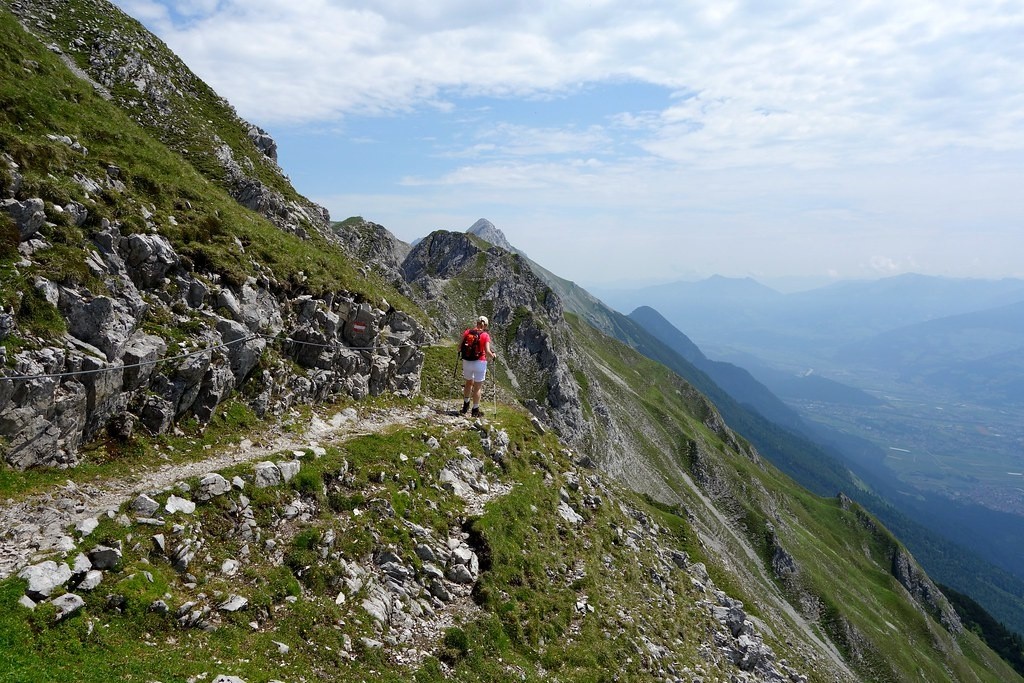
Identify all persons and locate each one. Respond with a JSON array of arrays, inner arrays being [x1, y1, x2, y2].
[[457, 316, 495, 418]]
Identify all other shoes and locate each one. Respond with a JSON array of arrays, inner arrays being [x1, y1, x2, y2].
[[472, 407, 484, 418], [460, 400, 470, 414]]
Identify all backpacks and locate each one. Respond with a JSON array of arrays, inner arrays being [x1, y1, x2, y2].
[[461, 330, 480, 361]]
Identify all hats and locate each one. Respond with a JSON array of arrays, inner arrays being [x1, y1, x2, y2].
[[477, 316, 488, 325]]
